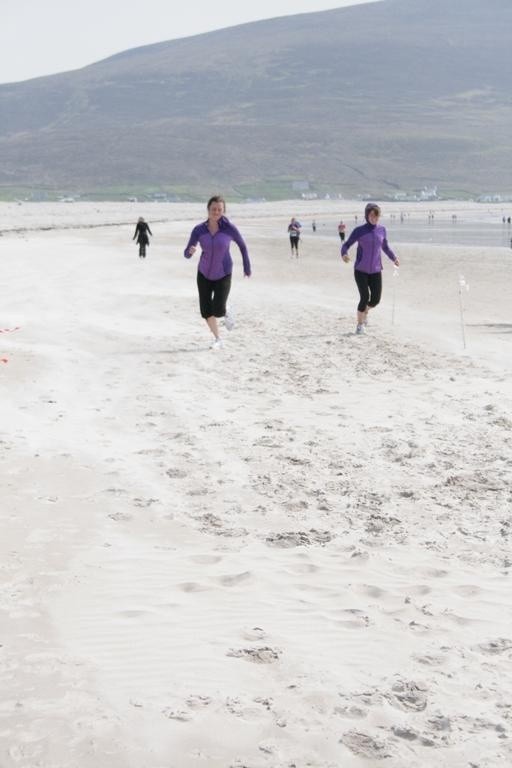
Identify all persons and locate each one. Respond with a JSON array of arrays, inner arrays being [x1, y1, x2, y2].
[[132, 216, 153, 258], [183, 194, 252, 352], [287, 217, 302, 260], [312, 219, 317, 232], [295, 219, 303, 244], [341, 203, 401, 335], [338, 221, 347, 244]]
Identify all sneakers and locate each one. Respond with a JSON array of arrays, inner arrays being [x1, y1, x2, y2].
[[208, 336, 224, 351], [355, 319, 370, 335]]
